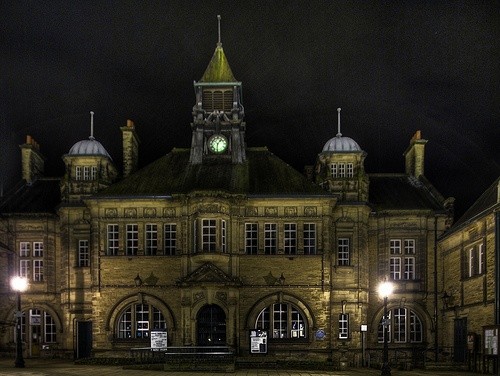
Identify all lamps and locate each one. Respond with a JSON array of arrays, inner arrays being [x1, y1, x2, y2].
[[134, 273, 142, 286], [441, 290, 451, 303], [279, 273, 285, 285]]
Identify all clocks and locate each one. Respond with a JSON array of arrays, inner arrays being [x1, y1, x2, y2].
[[207, 133, 228, 154]]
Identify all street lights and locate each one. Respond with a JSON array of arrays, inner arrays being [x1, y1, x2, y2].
[[12, 277, 26, 367], [379, 277, 392, 374]]
